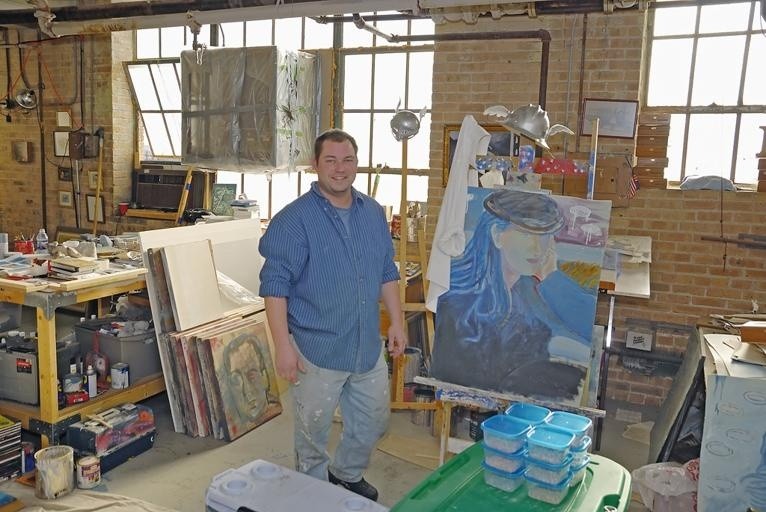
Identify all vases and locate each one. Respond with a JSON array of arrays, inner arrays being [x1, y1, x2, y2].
[[580, 98, 639, 139]]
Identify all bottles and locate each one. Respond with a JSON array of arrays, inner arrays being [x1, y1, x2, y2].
[[36, 228, 48, 254]]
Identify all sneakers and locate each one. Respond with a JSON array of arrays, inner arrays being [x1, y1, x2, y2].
[[326, 466, 379, 502]]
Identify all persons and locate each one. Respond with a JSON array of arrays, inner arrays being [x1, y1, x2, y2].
[[430, 190, 598, 403], [259, 128, 405, 502], [223, 333, 282, 439]]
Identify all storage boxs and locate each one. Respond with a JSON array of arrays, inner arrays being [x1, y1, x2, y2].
[[382, 432, 634, 512]]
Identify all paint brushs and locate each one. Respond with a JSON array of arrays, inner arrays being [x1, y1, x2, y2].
[[12, 232, 36, 241]]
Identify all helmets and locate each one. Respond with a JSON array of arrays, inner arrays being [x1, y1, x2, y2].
[[494, 103, 552, 152], [390, 109, 420, 142]]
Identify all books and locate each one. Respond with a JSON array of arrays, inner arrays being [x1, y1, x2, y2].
[[50, 256, 98, 276]]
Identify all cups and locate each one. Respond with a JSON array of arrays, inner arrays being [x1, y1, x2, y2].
[[118, 203, 129, 215]]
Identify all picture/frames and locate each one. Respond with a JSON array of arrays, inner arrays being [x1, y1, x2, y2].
[[85, 170, 105, 224], [442, 123, 511, 185], [53, 131, 73, 209]]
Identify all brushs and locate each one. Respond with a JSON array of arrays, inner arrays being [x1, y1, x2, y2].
[[85, 414, 113, 429]]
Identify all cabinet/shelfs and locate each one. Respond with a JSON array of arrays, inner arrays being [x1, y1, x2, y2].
[[2, 245, 167, 450]]
